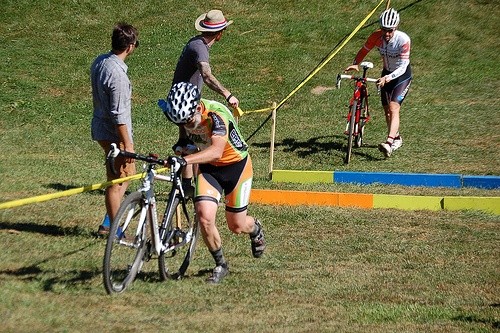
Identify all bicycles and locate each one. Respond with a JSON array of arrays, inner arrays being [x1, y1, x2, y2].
[[336, 61, 381, 164], [101, 143, 199, 297]]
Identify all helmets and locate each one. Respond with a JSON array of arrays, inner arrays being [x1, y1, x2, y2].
[[164, 82, 201, 125], [380, 8, 400, 27]]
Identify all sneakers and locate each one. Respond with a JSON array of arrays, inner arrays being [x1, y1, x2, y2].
[[249, 218, 265, 257], [378, 142, 392, 158], [391, 137, 402, 150], [207, 263, 229, 283]]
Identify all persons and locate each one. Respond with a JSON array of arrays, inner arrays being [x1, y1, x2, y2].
[[173, 10, 239, 200], [345, 8, 413, 158], [165, 82, 265, 283], [89, 22, 140, 239]]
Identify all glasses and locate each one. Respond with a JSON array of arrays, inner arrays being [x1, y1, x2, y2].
[[126, 40, 140, 48], [381, 28, 397, 33], [176, 119, 196, 127]]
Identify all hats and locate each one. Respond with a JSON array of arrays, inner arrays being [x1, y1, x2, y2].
[[195, 9, 233, 32]]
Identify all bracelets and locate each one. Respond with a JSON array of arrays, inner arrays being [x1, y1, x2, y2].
[[226, 94, 233, 102]]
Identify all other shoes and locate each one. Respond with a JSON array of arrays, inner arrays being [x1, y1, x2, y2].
[[114, 231, 137, 244], [97, 225, 110, 238]]
